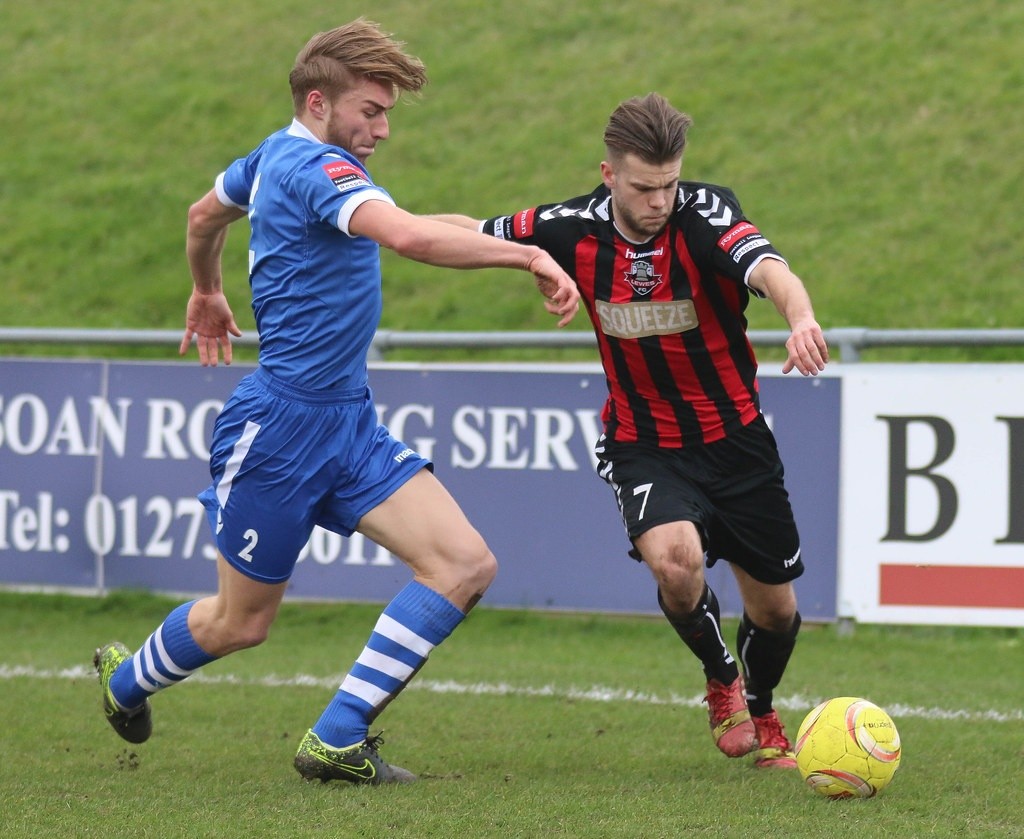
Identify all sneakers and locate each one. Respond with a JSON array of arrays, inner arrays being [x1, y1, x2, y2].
[[293, 728, 415, 784], [700, 673, 755, 758], [96, 642, 153, 744], [752, 709, 797, 770]]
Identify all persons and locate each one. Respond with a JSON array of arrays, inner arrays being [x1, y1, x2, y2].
[[416, 91, 830, 768], [93, 16, 581, 788]]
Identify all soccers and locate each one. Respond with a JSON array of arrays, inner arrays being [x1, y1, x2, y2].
[[794, 696, 903, 801]]
